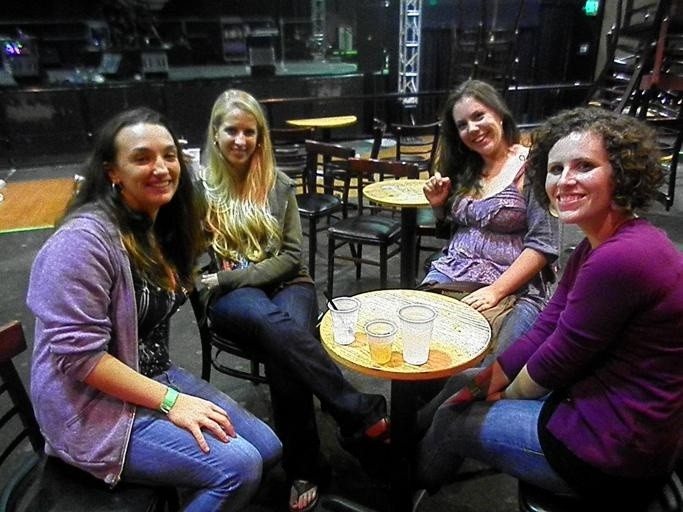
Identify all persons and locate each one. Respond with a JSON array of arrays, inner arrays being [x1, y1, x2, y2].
[[422, 79, 562, 368], [188, 89, 391, 511], [29, 106, 284, 512], [417, 107, 683, 496]]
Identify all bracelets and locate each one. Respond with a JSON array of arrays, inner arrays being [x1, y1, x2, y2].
[[465, 380, 486, 402], [157, 383, 180, 416]]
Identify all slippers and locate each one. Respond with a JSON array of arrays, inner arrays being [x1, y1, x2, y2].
[[287, 479, 319, 511]]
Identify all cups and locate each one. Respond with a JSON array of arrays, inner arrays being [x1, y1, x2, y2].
[[328, 294, 360, 347], [397, 302, 438, 367], [363, 320, 398, 366]]
